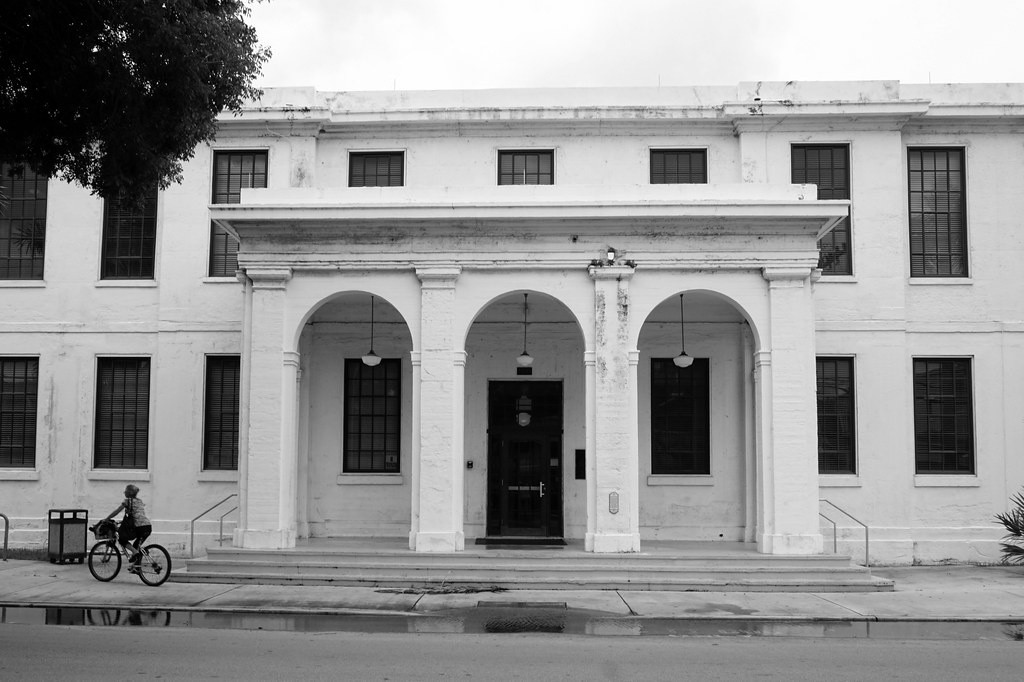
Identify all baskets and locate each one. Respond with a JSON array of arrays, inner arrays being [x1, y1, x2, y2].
[[95, 529, 109, 539]]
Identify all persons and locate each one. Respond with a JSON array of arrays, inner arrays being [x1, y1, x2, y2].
[[106, 484, 152, 571]]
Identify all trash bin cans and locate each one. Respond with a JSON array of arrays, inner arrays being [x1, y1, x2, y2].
[[47, 507, 90, 566]]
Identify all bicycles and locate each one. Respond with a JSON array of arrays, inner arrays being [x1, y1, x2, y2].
[[86, 518, 172, 588]]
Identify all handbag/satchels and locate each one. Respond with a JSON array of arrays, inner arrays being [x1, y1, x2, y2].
[[120, 497, 137, 540]]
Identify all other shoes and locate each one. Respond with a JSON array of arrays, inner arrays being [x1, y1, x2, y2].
[[128, 567, 139, 571], [129, 552, 140, 563]]
